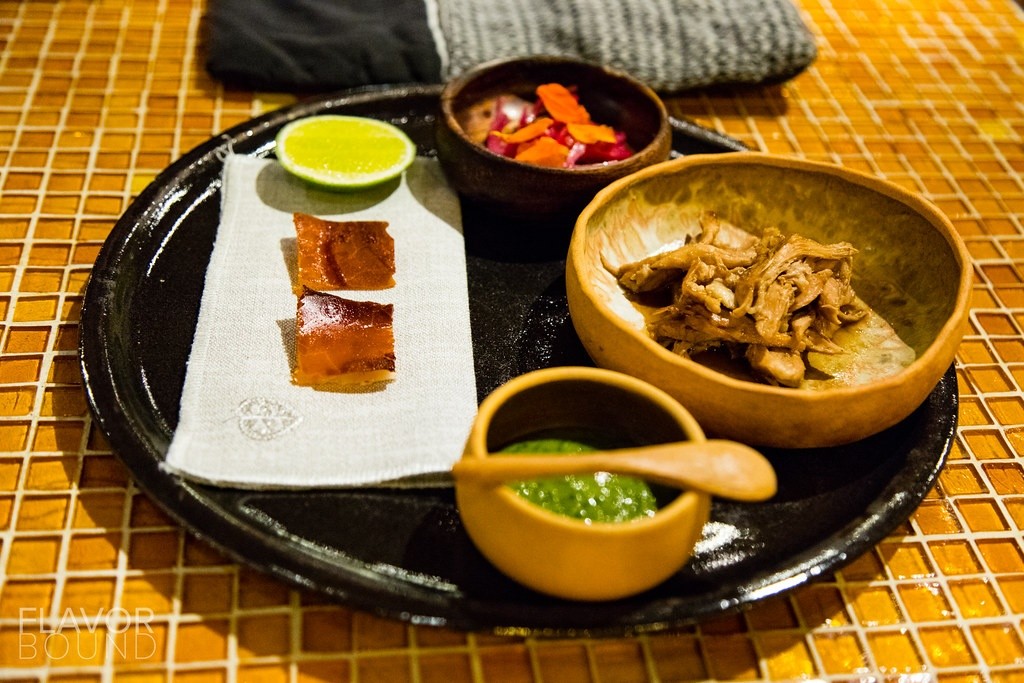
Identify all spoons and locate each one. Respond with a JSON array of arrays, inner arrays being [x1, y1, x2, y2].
[[452, 438, 777, 502]]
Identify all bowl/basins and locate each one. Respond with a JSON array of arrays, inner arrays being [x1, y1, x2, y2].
[[565, 154, 971, 451], [433, 54, 673, 220], [452, 368, 711, 602]]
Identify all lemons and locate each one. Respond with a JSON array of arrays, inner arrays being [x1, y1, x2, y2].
[[275, 114, 417, 189]]
